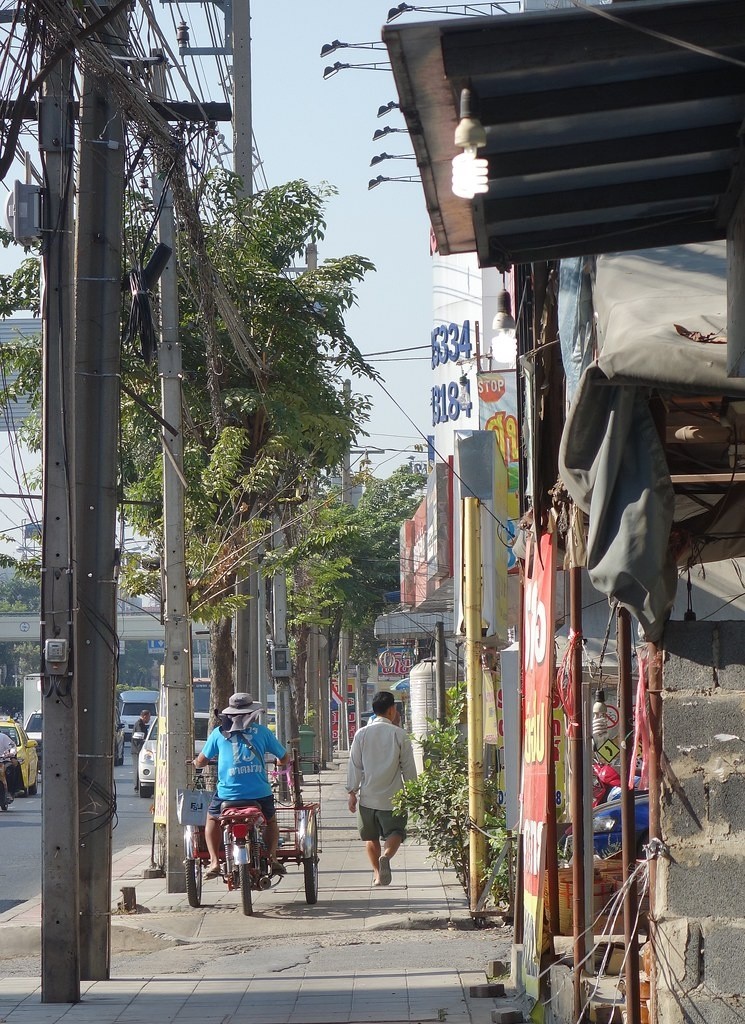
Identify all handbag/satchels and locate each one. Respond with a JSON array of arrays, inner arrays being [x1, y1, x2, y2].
[[176, 775, 215, 826]]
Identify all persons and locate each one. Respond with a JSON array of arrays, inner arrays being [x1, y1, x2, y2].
[[131, 710, 151, 792], [0, 732, 17, 802], [345, 691, 419, 887], [192, 693, 289, 880]]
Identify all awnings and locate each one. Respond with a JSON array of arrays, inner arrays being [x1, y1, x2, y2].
[[390, 678, 410, 691]]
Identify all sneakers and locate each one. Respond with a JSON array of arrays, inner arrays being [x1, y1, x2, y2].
[[373, 853, 392, 886]]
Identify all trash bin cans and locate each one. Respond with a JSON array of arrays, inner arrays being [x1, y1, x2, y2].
[[298, 731, 316, 775]]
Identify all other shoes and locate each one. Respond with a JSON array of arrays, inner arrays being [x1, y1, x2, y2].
[[134, 787, 138, 791]]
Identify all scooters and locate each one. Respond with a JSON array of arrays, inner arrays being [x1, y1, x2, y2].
[[0, 742, 25, 811]]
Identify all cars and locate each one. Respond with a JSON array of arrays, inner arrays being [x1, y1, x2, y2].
[[133, 711, 209, 797], [25, 708, 42, 773], [113, 706, 123, 765], [265, 708, 277, 736], [0, 715, 39, 795]]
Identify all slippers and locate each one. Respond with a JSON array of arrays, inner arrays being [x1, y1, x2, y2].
[[269, 857, 288, 875], [204, 866, 219, 880]]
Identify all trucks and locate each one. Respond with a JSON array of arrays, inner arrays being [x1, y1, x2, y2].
[[118, 691, 159, 741]]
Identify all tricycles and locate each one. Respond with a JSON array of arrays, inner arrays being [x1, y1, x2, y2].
[[180, 746, 321, 916]]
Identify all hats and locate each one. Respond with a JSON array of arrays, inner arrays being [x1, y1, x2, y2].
[[222, 692, 263, 714]]
